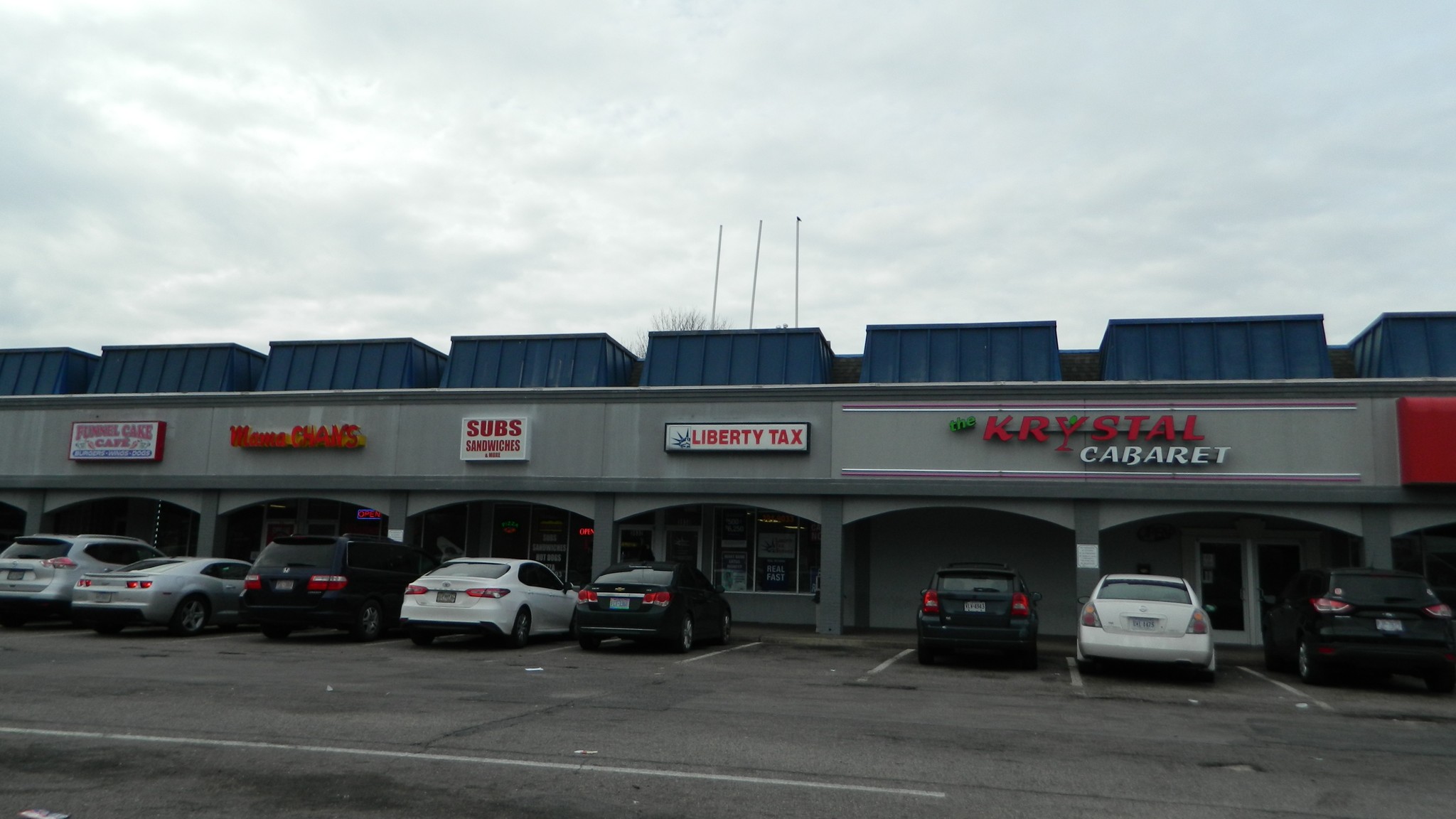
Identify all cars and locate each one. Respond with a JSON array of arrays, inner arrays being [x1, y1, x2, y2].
[[570, 561, 733, 652], [400, 557, 579, 647], [1268, 568, 1456, 688], [71, 557, 253, 637], [1075, 574, 1221, 679]]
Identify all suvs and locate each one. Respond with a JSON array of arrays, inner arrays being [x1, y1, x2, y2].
[[240, 530, 444, 644], [0, 534, 173, 629], [915, 561, 1043, 668]]
